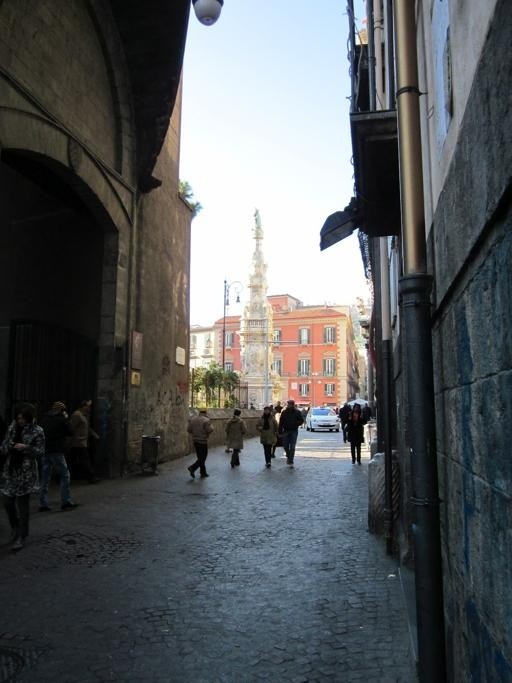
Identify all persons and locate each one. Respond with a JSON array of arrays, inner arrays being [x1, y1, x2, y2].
[[278, 399, 305, 469], [255, 405, 280, 469], [185, 407, 216, 478], [224, 407, 250, 469], [0, 402, 48, 552], [266, 400, 310, 431], [37, 397, 82, 512], [69, 397, 104, 486], [331, 402, 374, 443], [344, 403, 367, 466]]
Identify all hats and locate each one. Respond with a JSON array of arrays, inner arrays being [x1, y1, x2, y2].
[[49, 399, 66, 415]]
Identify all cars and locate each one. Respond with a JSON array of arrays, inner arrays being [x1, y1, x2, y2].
[[304, 406, 340, 433]]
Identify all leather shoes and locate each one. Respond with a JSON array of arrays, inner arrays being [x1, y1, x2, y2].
[[9, 527, 26, 550], [39, 507, 54, 512], [61, 499, 80, 511], [188, 466, 195, 478], [200, 472, 209, 476]]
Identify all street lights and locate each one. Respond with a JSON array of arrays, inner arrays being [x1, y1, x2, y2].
[[222, 278, 245, 370], [190, 0, 225, 26]]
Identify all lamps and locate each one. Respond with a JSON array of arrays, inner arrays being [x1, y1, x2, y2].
[[192, 0, 223, 25]]
